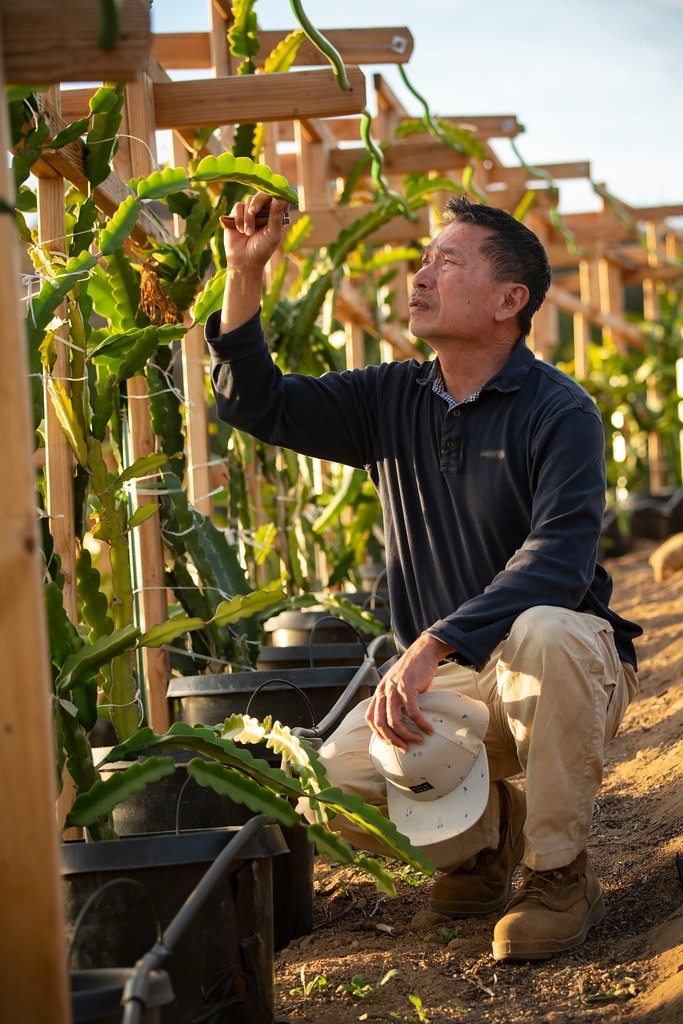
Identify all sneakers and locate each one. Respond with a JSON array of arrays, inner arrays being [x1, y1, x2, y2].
[[491, 848, 607, 961], [427, 779, 527, 918]]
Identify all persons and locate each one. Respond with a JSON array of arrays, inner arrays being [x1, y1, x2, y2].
[[204, 191, 643, 962]]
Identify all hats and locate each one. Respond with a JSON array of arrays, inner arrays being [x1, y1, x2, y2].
[[369, 690, 489, 846]]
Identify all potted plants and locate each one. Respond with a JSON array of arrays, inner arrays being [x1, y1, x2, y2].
[[9, 73, 299, 1024], [139, 370, 392, 670], [154, 471, 381, 773], [71, 256, 422, 948], [260, 472, 386, 647], [315, 545, 377, 612]]
